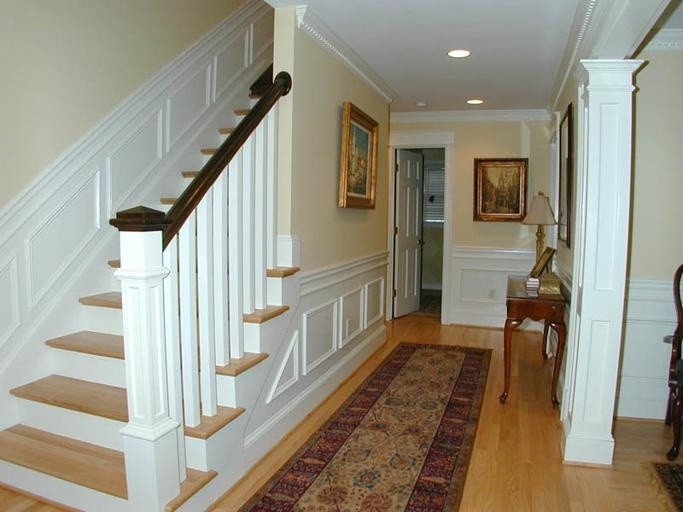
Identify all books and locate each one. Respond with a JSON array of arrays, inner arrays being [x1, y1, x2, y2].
[[524, 277, 539, 297]]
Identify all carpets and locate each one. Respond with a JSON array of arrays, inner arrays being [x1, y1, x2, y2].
[[235, 339, 493, 511], [643, 461, 683, 511]]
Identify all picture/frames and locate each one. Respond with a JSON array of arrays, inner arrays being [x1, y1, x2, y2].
[[529, 246, 555, 279], [556, 103, 573, 249], [337, 99, 378, 211], [472, 157, 528, 223]]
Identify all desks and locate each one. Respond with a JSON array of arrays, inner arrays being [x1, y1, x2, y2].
[[499, 272, 566, 410]]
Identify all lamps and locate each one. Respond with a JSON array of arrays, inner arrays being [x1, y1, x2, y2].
[[521, 190, 556, 263]]
